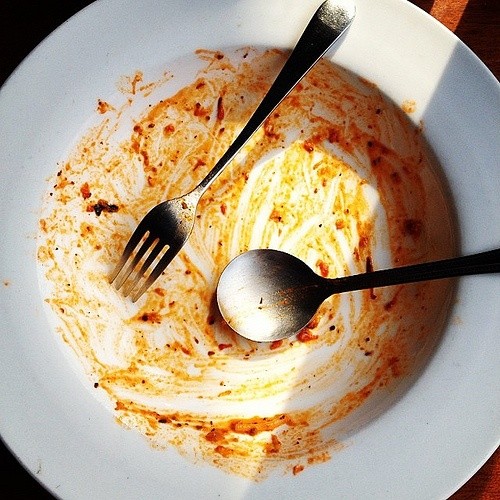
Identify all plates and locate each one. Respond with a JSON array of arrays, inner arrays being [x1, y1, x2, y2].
[[0, 0, 500, 500]]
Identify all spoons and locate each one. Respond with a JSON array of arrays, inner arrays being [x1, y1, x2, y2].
[[216, 249, 500, 342]]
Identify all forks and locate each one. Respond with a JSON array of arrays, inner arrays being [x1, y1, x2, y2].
[[109, 0, 357, 304]]
